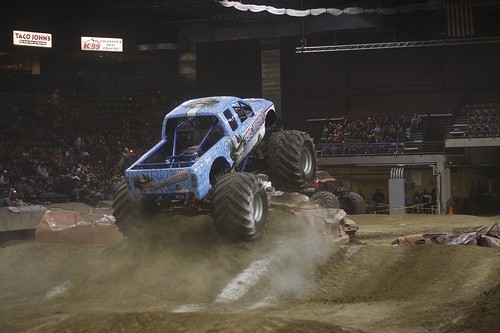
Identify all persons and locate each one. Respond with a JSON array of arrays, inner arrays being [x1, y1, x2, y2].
[[0, 87, 188, 208], [357, 188, 386, 214], [414, 188, 437, 214], [320, 110, 423, 154]]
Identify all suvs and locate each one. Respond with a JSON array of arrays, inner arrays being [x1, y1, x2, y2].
[[304, 170, 366, 217], [111, 96, 318, 242]]
